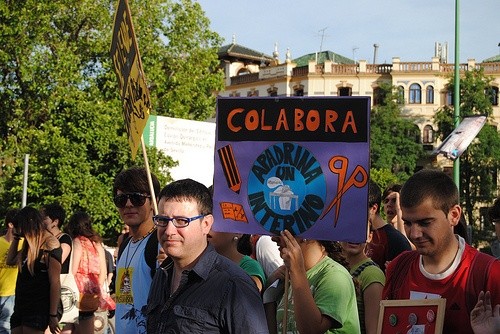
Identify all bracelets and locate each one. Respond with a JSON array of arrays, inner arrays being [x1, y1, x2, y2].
[[49, 314, 57, 317]]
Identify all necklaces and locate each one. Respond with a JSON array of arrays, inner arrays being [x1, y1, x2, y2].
[[55, 232, 61, 237], [125, 222, 158, 276]]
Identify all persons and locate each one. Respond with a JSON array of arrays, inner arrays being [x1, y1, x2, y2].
[[248, 185, 500, 334], [0, 209, 24, 334], [145, 179, 269, 334], [42, 203, 80, 334], [273, 230, 361, 334], [206, 183, 266, 295], [113, 166, 162, 334], [381, 168, 500, 334], [65, 208, 132, 334], [6, 206, 63, 334]]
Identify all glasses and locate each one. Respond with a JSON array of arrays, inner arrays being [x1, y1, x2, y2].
[[152, 212, 212, 228], [113, 193, 150, 209]]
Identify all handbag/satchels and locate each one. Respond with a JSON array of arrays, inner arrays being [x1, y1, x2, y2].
[[75, 292, 99, 311], [59, 273, 80, 325]]
